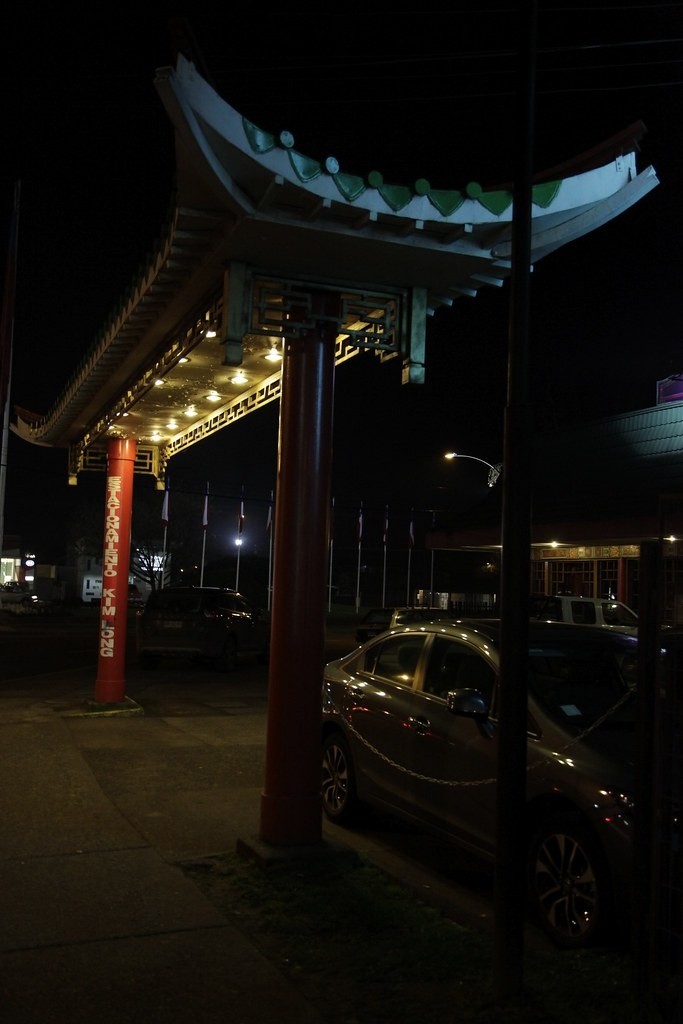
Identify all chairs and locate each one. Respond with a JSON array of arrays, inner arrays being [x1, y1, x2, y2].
[[397, 647, 420, 670]]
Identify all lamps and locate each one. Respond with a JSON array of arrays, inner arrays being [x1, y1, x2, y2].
[[442, 451, 495, 473]]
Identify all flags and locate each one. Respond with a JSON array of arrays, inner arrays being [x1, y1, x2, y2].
[[200, 484, 211, 529], [407, 510, 416, 549], [353, 512, 365, 547], [236, 486, 246, 532], [265, 492, 273, 537], [162, 475, 173, 529], [382, 510, 390, 544], [329, 504, 336, 544]]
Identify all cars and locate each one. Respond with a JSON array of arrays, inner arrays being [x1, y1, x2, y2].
[[134, 586, 272, 673], [0, 581, 49, 611], [320, 617, 683, 956]]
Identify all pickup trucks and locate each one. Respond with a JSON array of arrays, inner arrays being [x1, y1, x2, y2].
[[531, 594, 670, 642]]
